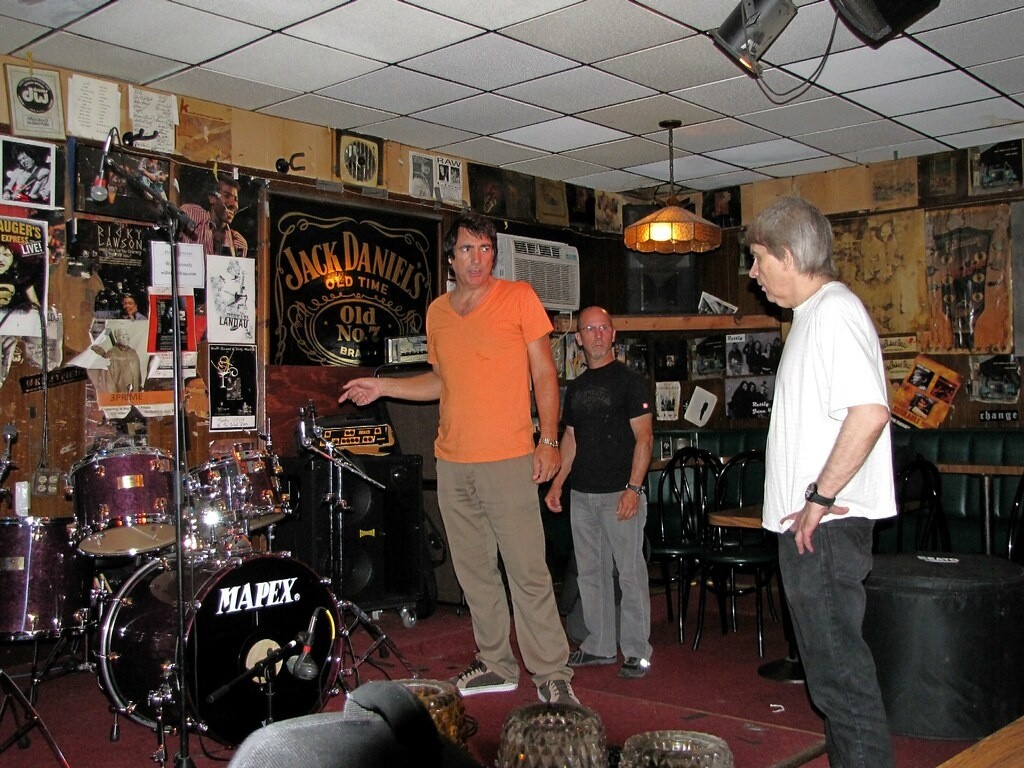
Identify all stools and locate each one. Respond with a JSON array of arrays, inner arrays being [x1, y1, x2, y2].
[[862, 552, 1024, 740]]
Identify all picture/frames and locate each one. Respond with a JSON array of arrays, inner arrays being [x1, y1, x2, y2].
[[2, 62, 67, 143], [73, 143, 170, 226]]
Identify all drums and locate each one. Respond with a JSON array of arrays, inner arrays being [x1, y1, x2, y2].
[[0, 516, 83, 642], [98, 549, 344, 749], [182, 449, 290, 533], [70, 446, 178, 558]]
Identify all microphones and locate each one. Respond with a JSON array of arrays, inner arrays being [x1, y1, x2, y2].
[[288, 608, 321, 681], [91, 131, 114, 201]]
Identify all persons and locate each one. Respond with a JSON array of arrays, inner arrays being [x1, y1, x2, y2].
[[4, 142, 51, 205], [910, 368, 934, 418], [119, 292, 147, 319], [728, 335, 782, 374], [412, 158, 431, 196], [545, 306, 654, 678], [667, 357, 673, 367], [0, 243, 42, 309], [160, 304, 174, 335], [337, 216, 580, 707], [139, 152, 174, 225], [180, 173, 248, 258], [440, 165, 460, 182], [730, 381, 770, 421], [743, 198, 899, 768], [102, 327, 141, 392], [568, 339, 588, 378]]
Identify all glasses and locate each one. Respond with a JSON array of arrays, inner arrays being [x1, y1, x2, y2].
[[580, 325, 613, 332]]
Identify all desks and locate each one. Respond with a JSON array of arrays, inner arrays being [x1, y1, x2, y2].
[[709, 501, 809, 685], [933, 464, 1024, 559]]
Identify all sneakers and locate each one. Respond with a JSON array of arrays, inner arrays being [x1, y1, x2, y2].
[[566, 648, 617, 667], [537, 680, 581, 706], [448, 660, 518, 697], [618, 657, 651, 679]]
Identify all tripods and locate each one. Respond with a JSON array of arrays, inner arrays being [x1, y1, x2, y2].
[[309, 404, 420, 684]]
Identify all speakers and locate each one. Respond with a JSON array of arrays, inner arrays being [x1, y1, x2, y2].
[[294, 451, 424, 613]]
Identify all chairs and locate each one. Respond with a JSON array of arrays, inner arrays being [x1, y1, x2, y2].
[[644, 444, 1024, 659]]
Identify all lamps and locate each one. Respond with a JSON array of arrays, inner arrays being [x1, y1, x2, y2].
[[623, 119, 723, 254], [707, 0, 798, 75]]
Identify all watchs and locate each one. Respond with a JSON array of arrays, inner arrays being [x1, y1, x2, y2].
[[626, 482, 645, 496], [538, 438, 559, 448], [805, 482, 836, 507]]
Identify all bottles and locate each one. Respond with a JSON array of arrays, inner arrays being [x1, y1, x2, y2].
[[294, 646, 319, 680]]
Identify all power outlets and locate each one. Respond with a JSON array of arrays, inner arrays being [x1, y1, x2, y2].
[[31, 470, 60, 498]]
